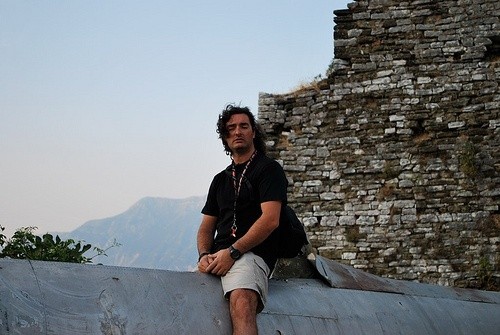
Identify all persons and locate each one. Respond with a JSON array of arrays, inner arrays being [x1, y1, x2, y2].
[[196, 104, 289, 335]]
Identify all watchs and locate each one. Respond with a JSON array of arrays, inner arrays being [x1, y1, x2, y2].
[[229, 245, 242, 260]]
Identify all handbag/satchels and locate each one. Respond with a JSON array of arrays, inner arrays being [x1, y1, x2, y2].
[[279, 207, 307, 260]]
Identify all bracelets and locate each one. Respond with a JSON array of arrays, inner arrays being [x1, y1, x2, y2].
[[198, 253, 210, 261]]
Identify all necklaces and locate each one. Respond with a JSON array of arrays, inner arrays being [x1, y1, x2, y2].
[[230, 151, 258, 237]]
